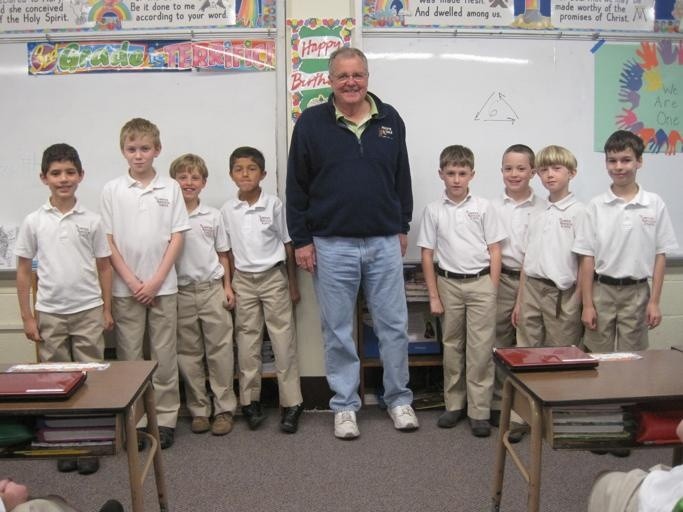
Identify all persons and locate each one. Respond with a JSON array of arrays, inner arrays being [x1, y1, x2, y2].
[[98, 119, 192, 449], [169, 154, 235, 436], [218, 147, 301, 433], [490, 143, 545, 426], [572, 129, 679, 353], [14, 144, 113, 477], [0, 473, 124, 512], [588, 418, 683, 512], [421, 143, 510, 437], [286, 47, 421, 441], [509, 146, 596, 443]]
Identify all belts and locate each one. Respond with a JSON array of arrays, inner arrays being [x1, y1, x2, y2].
[[501, 268, 520, 276], [436, 263, 490, 279], [594, 274, 647, 285], [535, 278, 556, 287]]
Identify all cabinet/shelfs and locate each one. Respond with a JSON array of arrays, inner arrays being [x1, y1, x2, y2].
[[357, 267, 445, 407]]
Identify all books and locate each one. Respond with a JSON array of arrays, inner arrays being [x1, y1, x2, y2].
[[401, 263, 432, 305]]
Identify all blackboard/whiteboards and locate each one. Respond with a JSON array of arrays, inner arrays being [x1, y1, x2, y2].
[[0, 29, 278, 280], [361, 29, 683, 265]]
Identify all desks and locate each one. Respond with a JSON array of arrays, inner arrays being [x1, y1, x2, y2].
[[490, 345, 683, 511], [3, 359, 171, 512]]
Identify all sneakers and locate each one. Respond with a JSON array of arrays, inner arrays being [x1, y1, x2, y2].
[[438, 409, 465, 428], [57, 458, 98, 475], [334, 411, 360, 438], [280, 405, 303, 433], [212, 413, 234, 434], [99, 499, 123, 512], [470, 419, 491, 437], [242, 401, 267, 430], [158, 426, 175, 449], [387, 405, 420, 429], [191, 417, 210, 433], [125, 428, 147, 451], [509, 421, 530, 443]]
